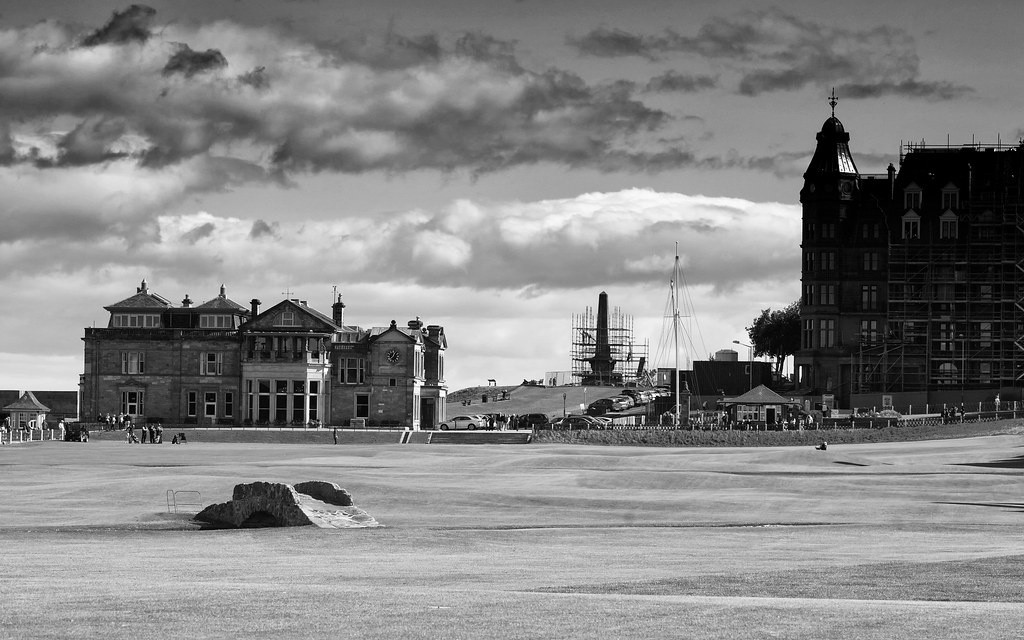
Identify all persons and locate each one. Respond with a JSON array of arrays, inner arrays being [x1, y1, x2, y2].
[[171, 433, 180, 444], [487, 412, 519, 432], [943, 405, 965, 424], [80, 429, 89, 443], [96, 412, 163, 444], [333, 426, 338, 445]]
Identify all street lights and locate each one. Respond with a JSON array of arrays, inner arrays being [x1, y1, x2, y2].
[[563, 392, 566, 418]]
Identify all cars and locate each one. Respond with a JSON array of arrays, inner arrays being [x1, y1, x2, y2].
[[517, 413, 549, 429], [549, 415, 614, 430], [588, 388, 672, 412], [437, 414, 502, 430]]
